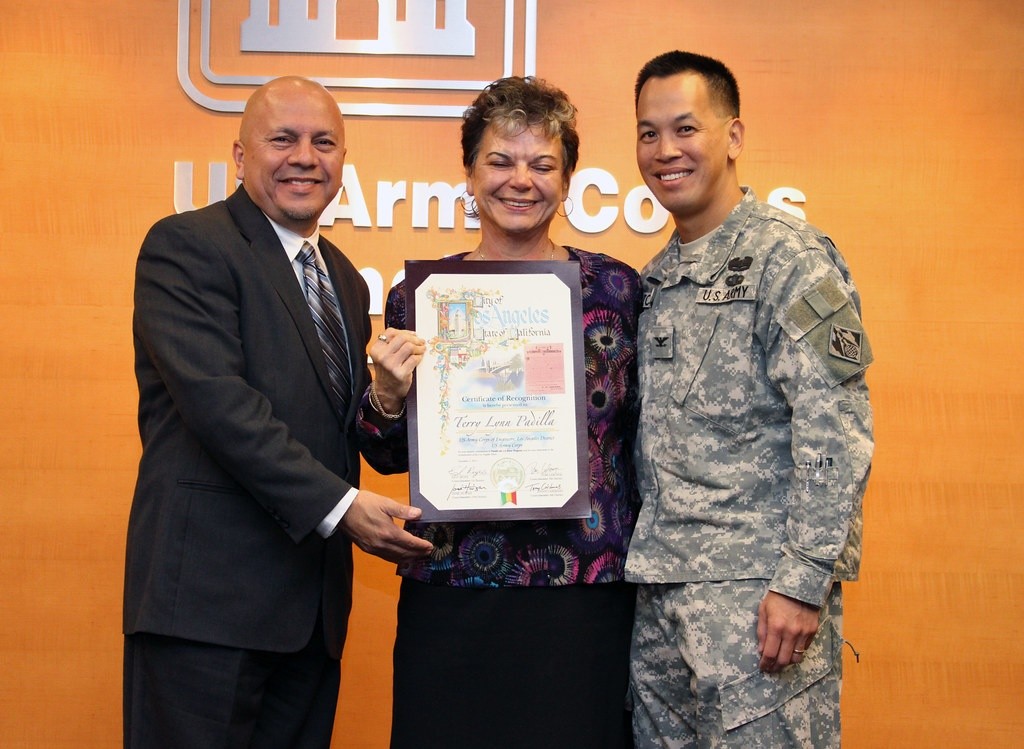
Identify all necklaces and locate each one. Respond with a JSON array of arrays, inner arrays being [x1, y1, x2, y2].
[[479, 236, 556, 260]]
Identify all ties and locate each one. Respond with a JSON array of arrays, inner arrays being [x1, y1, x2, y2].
[[294, 241, 352, 433]]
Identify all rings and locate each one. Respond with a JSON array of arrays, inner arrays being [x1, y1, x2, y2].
[[793, 648, 806, 655], [377, 334, 390, 345]]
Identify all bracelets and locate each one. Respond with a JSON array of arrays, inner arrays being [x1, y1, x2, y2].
[[369, 379, 407, 420]]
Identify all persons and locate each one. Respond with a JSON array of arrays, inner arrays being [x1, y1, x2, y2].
[[117, 75, 437, 749], [624, 48, 881, 749], [353, 72, 648, 748]]
